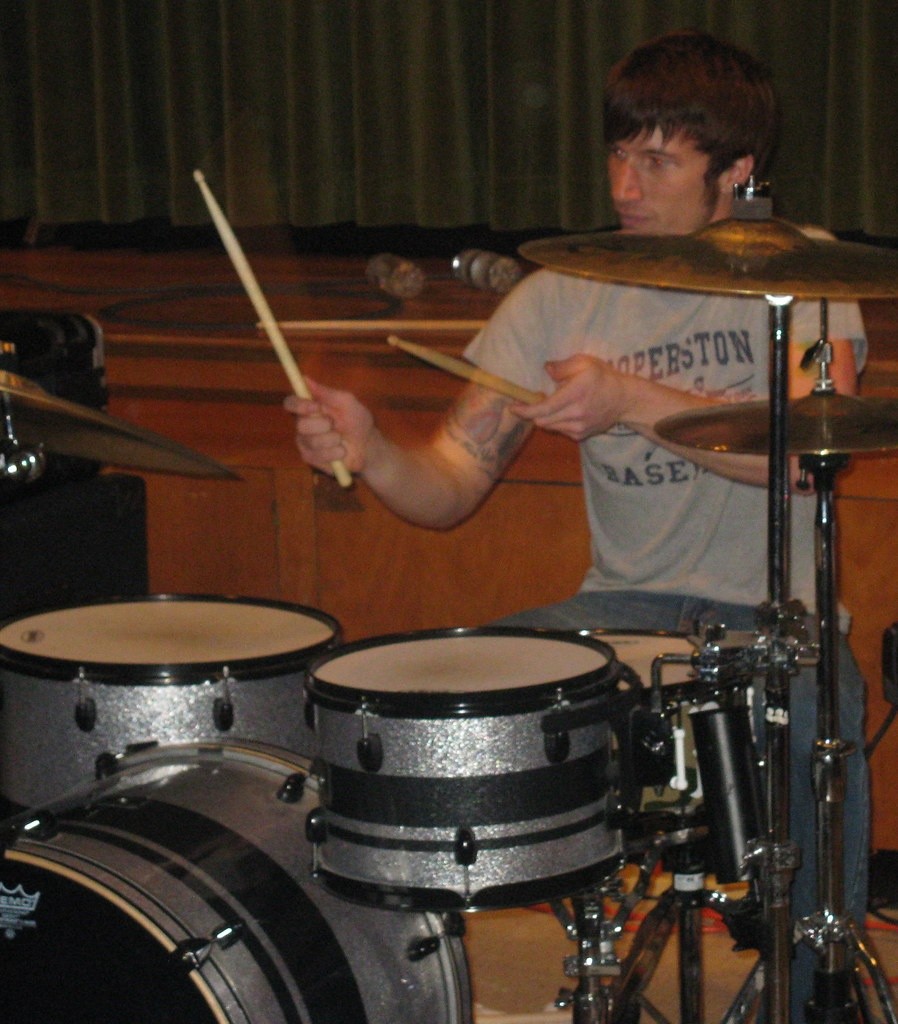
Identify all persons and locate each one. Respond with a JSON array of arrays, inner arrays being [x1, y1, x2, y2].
[[284, 35, 868, 1024]]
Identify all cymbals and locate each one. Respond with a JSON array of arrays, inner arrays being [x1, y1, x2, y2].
[[652, 389, 897, 457], [513, 217, 896, 300], [0, 366, 246, 482]]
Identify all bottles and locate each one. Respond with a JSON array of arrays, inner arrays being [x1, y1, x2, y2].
[[366, 255, 424, 298], [452, 250, 522, 294]]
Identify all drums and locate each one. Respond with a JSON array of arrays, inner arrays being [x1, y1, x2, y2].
[[1, 593, 348, 815], [302, 625, 631, 916], [2, 738, 475, 1024], [589, 620, 783, 865]]
